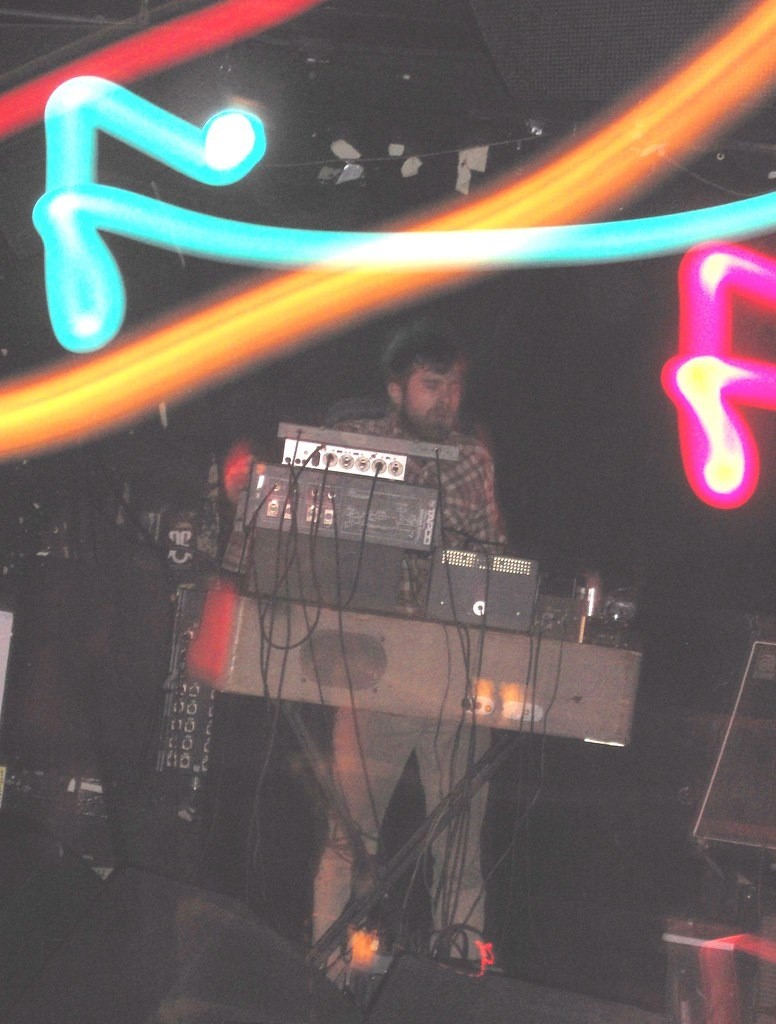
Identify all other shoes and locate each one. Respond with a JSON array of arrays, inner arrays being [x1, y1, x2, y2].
[[316, 943, 394, 978], [429, 926, 504, 976]]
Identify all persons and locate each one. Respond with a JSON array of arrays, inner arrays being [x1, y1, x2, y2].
[[309, 322, 510, 974]]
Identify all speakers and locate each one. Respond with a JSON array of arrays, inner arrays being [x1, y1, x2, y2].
[[0, 814, 678, 1024], [694, 641, 776, 850]]
